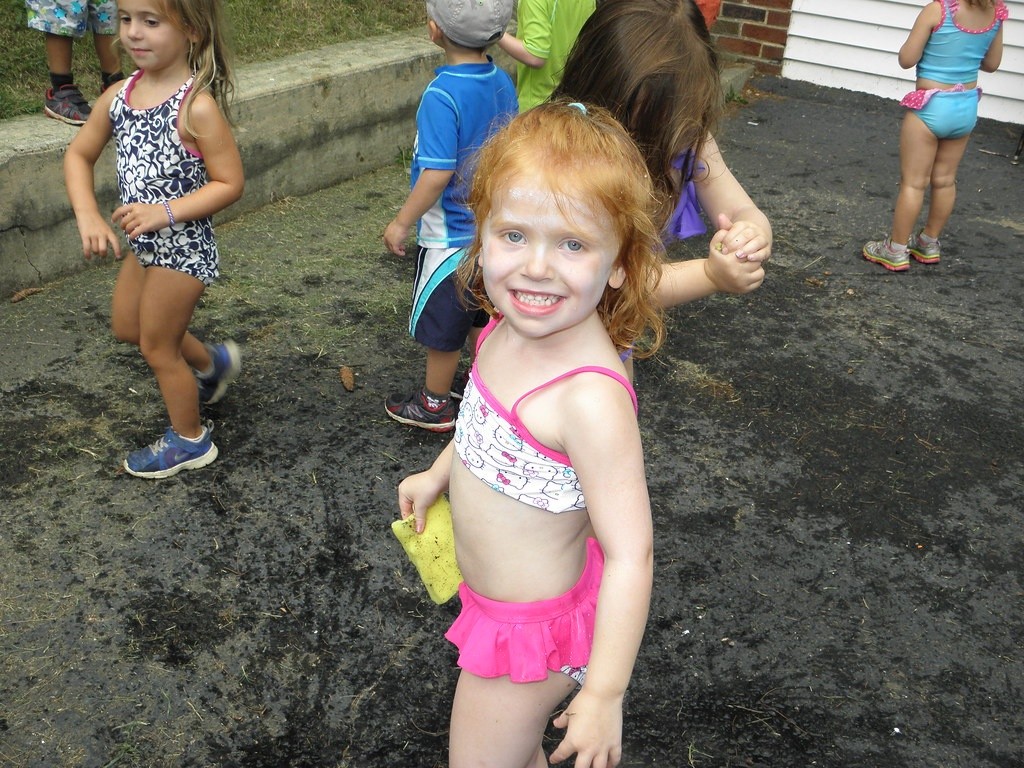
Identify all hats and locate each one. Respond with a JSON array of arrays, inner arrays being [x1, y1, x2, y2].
[[424, 0, 513, 48]]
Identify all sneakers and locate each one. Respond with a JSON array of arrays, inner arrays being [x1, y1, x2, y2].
[[194, 339, 241, 404], [905, 228, 940, 263], [101, 71, 124, 92], [45, 84, 92, 125], [384, 389, 455, 432], [449, 369, 471, 399], [124, 419, 219, 478], [862, 237, 911, 271]]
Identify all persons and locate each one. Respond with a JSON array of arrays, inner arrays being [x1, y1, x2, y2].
[[382, 0, 521, 432], [550, 0, 774, 388], [493, 0, 597, 115], [61, 2, 245, 478], [395, 94, 665, 768], [25, 0, 125, 126], [862, 0, 1010, 272]]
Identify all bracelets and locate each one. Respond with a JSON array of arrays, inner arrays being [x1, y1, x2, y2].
[[163, 201, 175, 226]]
